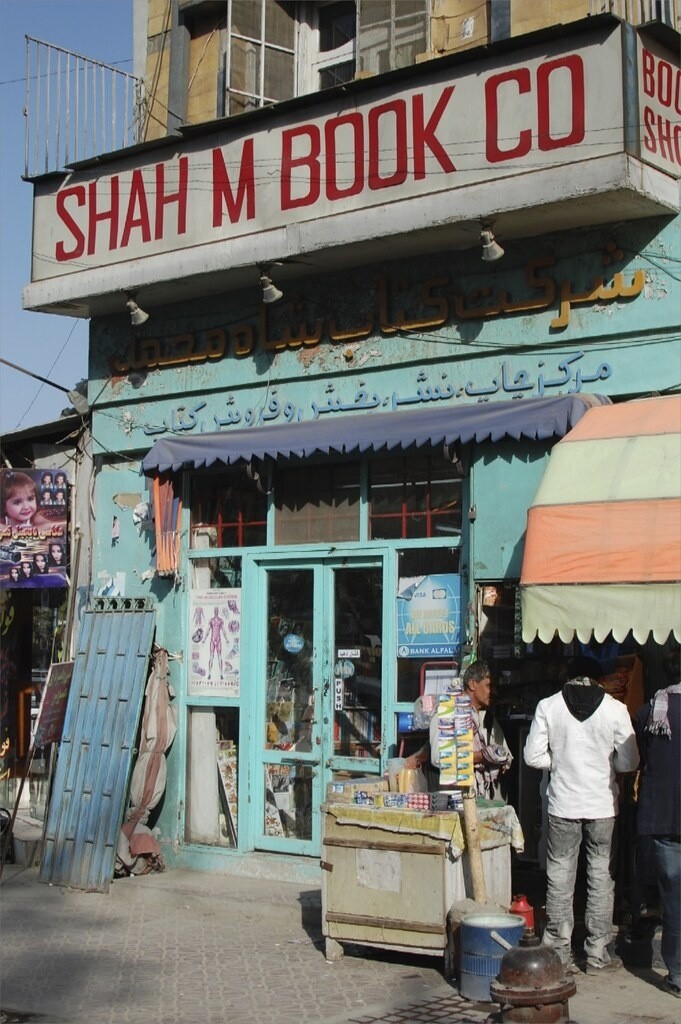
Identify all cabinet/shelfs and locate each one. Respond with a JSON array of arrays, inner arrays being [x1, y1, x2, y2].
[[320, 803, 524, 964]]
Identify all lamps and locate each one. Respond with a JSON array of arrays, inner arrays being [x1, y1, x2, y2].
[[124, 291, 150, 325], [260, 270, 284, 304], [478, 218, 506, 263]]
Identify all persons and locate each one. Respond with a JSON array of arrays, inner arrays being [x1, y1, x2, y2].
[[0, 469, 67, 583], [428, 660, 514, 804], [634, 648, 681, 998]]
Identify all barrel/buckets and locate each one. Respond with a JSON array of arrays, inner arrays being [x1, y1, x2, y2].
[[459, 914, 525, 1001]]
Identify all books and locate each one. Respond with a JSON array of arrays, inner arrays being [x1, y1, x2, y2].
[[334, 711, 381, 741], [335, 749, 373, 758], [343, 682, 369, 709], [351, 645, 382, 673]]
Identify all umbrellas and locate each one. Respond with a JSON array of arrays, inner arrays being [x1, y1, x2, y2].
[[114, 649, 178, 876]]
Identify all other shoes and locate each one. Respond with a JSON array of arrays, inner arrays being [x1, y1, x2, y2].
[[586, 958, 623, 975], [625, 959, 651, 968], [564, 966, 580, 976], [662, 975, 681, 997]]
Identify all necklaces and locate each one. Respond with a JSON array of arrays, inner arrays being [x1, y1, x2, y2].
[[522, 656, 640, 976]]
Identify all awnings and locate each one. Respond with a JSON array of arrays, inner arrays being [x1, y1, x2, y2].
[[519, 394, 681, 646]]
